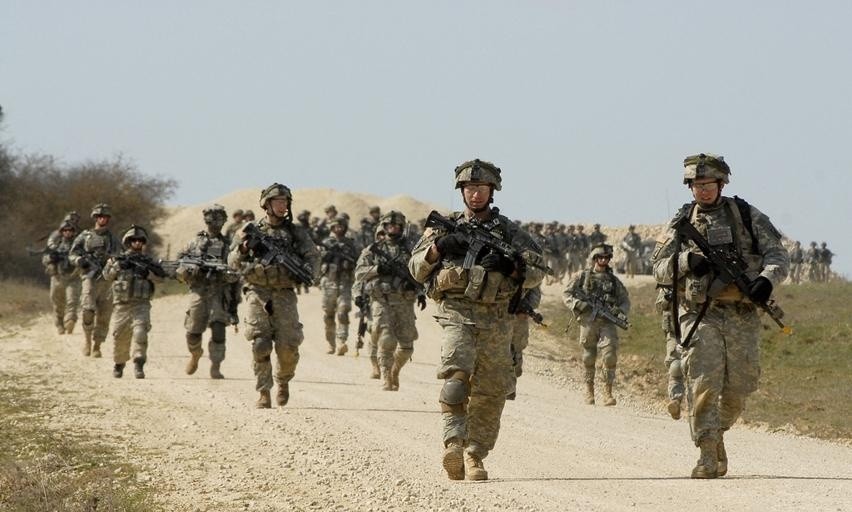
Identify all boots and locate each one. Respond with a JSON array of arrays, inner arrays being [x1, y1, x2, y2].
[[691, 435, 717, 479], [58, 318, 74, 334], [210, 360, 224, 379], [371, 366, 399, 391], [463, 449, 487, 480], [443, 442, 465, 480], [327, 343, 348, 356], [717, 430, 727, 476], [603, 383, 616, 406], [668, 397, 680, 420], [114, 362, 126, 378], [277, 379, 289, 406], [186, 347, 203, 375], [546, 272, 579, 286], [585, 383, 595, 405], [625, 273, 636, 279], [84, 337, 101, 358], [134, 359, 145, 379], [257, 389, 271, 409]]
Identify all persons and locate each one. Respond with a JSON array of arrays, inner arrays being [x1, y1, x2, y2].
[[788, 240, 806, 284], [620, 225, 644, 276], [806, 241, 820, 281], [651, 150, 789, 480], [407, 160, 547, 481], [563, 245, 633, 409], [653, 284, 694, 422], [816, 240, 834, 280], [228, 180, 324, 408], [177, 204, 427, 390], [44, 202, 155, 379], [513, 219, 607, 380]]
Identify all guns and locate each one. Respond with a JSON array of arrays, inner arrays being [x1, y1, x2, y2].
[[569, 285, 632, 330], [670, 217, 785, 329], [113, 253, 169, 279], [44, 246, 68, 268], [423, 210, 555, 276], [242, 223, 315, 287], [71, 244, 104, 280], [366, 244, 428, 294], [313, 237, 357, 265], [157, 255, 238, 282]]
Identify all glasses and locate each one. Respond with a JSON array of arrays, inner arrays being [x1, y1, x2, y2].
[[463, 183, 492, 191], [691, 182, 718, 192]]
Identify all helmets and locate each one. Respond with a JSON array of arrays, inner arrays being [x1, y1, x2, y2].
[[683, 153, 731, 184], [121, 224, 147, 249], [59, 211, 81, 233], [454, 159, 503, 190], [514, 221, 584, 232], [380, 210, 406, 227], [260, 182, 292, 206], [628, 225, 635, 232], [593, 224, 601, 230], [233, 209, 254, 220], [590, 243, 613, 262], [90, 202, 113, 219], [203, 204, 227, 226], [298, 206, 384, 239], [794, 241, 826, 248]]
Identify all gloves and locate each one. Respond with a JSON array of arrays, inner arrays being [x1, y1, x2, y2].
[[323, 250, 336, 262], [576, 300, 593, 313], [480, 252, 516, 278], [50, 253, 89, 269], [119, 260, 149, 276], [687, 250, 707, 277], [610, 306, 624, 316], [436, 234, 461, 255], [417, 294, 426, 311], [246, 236, 262, 252], [748, 276, 772, 302], [379, 262, 395, 275]]
